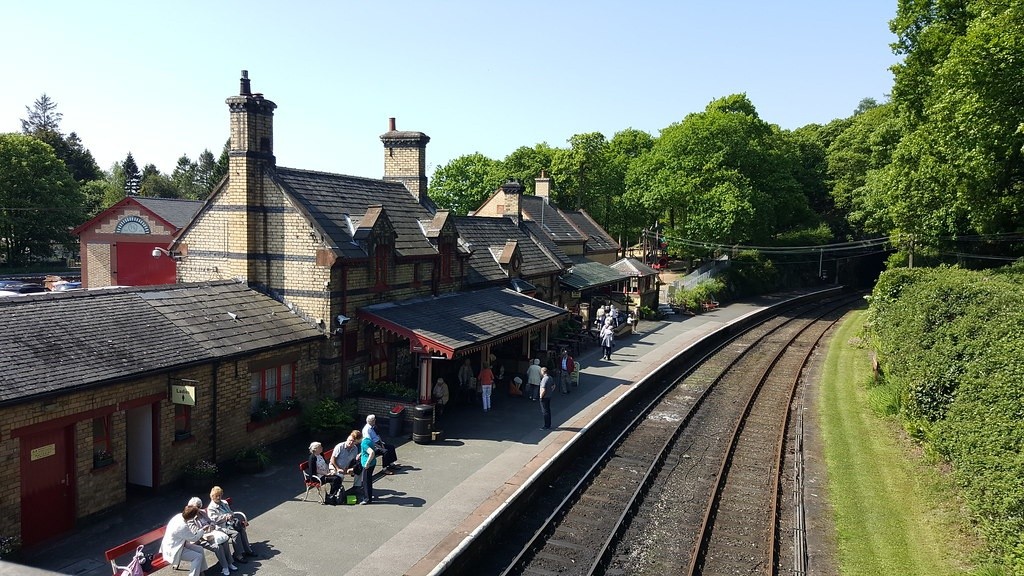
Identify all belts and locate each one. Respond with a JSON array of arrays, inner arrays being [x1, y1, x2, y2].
[[561, 369, 567, 371]]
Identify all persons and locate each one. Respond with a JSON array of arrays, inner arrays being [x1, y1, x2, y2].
[[352, 430, 376, 504], [559, 350, 574, 395], [309, 442, 343, 504], [362, 414, 402, 475], [434, 378, 448, 405], [600, 321, 616, 360], [527, 359, 542, 401], [458, 359, 473, 386], [478, 362, 494, 412], [539, 367, 555, 430], [158, 485, 258, 576], [627, 306, 640, 332], [646, 252, 666, 271], [490, 354, 504, 381], [596, 304, 627, 329], [329, 435, 364, 495], [520, 359, 535, 398], [684, 300, 689, 313]]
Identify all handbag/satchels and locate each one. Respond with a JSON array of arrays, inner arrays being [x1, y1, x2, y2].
[[325, 493, 337, 506], [202, 529, 229, 545], [121, 545, 153, 576], [600, 338, 606, 346], [594, 320, 597, 324], [337, 485, 346, 504]]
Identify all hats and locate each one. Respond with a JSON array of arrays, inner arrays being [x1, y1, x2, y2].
[[534, 359, 540, 364], [437, 378, 444, 382]]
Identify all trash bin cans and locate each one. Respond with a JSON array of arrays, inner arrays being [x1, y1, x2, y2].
[[389, 407, 406, 437], [413, 405, 434, 444]]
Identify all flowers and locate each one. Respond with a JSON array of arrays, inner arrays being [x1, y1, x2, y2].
[[93, 449, 113, 461], [184, 459, 218, 478]]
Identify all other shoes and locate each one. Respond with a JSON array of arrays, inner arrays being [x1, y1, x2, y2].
[[222, 568, 230, 575], [359, 500, 368, 505], [382, 469, 393, 474], [483, 409, 487, 413], [487, 408, 491, 411], [247, 552, 258, 556], [228, 564, 238, 570], [354, 486, 364, 490], [234, 555, 247, 563], [539, 427, 549, 430], [529, 396, 537, 400], [390, 464, 401, 470], [561, 393, 567, 396]]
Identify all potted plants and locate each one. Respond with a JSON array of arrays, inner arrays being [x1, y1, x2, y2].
[[234, 440, 274, 474], [307, 398, 345, 444]]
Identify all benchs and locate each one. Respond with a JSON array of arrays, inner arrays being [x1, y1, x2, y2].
[[300, 449, 355, 505], [700, 300, 720, 312], [105, 498, 249, 576]]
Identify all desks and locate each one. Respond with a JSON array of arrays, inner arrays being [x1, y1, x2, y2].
[[548, 333, 593, 358]]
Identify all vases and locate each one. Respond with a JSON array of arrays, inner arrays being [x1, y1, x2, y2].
[[94, 458, 113, 469], [176, 431, 190, 441], [187, 475, 216, 489]]
